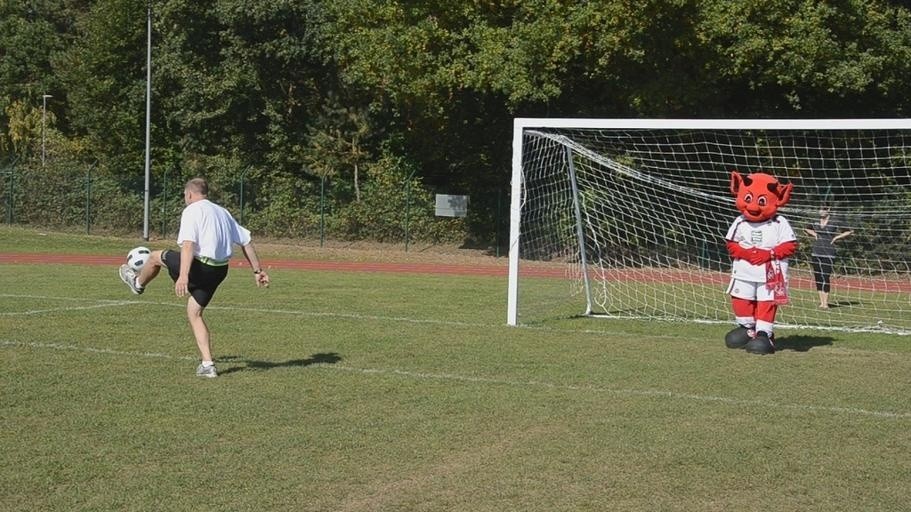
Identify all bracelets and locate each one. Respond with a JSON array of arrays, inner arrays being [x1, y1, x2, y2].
[[253, 270, 264, 274]]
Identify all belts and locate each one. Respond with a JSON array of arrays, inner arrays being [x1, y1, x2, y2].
[[197, 257, 229, 266]]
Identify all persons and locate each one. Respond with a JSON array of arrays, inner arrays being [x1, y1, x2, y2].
[[803, 200, 851, 309], [119, 178, 270, 377]]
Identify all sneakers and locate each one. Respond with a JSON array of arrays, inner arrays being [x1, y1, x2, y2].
[[119, 264, 146, 295], [196, 364, 218, 378]]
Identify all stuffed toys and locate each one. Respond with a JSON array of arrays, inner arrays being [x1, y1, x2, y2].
[[726, 170, 798, 355]]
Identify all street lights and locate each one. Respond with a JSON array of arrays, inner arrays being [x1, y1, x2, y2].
[[41, 93, 57, 165]]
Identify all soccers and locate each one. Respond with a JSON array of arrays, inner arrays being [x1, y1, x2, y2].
[[128, 246, 151, 272]]
